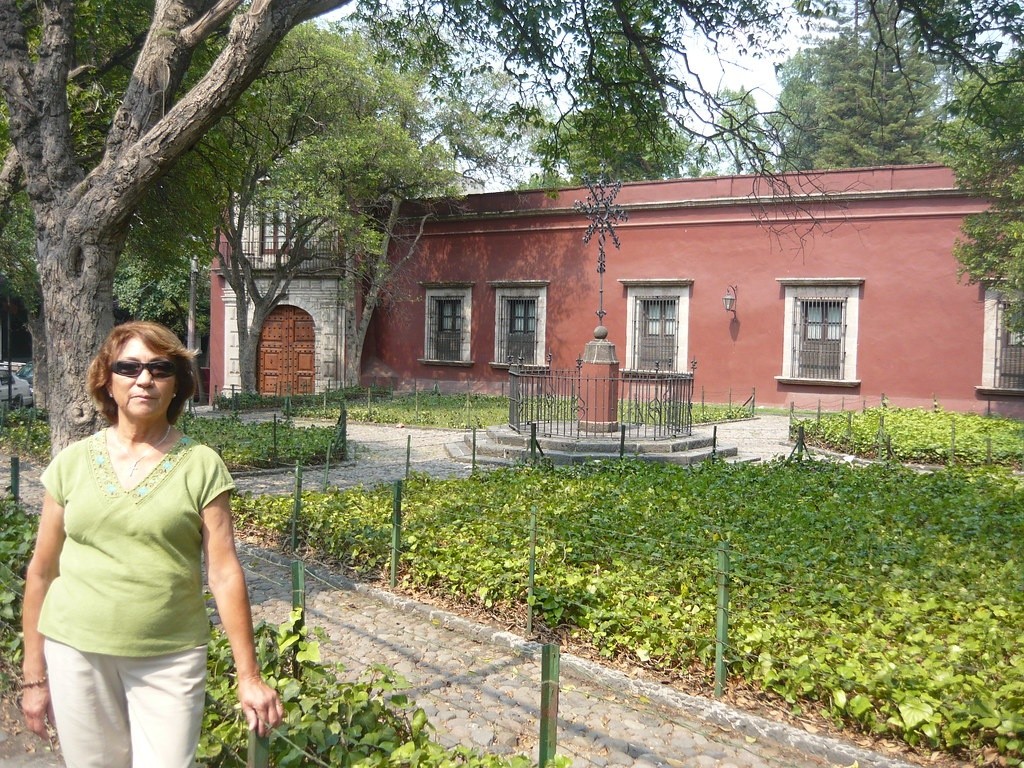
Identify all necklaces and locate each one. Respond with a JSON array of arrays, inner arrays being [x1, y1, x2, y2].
[[114, 426, 170, 477]]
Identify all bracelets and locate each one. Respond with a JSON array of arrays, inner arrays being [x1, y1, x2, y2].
[[18, 675, 46, 688]]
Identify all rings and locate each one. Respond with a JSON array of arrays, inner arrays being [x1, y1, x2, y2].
[[277, 704, 284, 710]]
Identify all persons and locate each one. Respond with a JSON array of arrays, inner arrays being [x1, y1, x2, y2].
[[21, 321, 284, 768]]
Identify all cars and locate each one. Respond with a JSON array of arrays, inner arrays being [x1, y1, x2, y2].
[[0, 361, 34, 386], [0, 368, 30, 408]]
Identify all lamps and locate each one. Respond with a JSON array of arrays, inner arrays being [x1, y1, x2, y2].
[[724, 285, 737, 322]]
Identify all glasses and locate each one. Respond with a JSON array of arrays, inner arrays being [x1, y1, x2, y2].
[[111, 360, 176, 378]]
